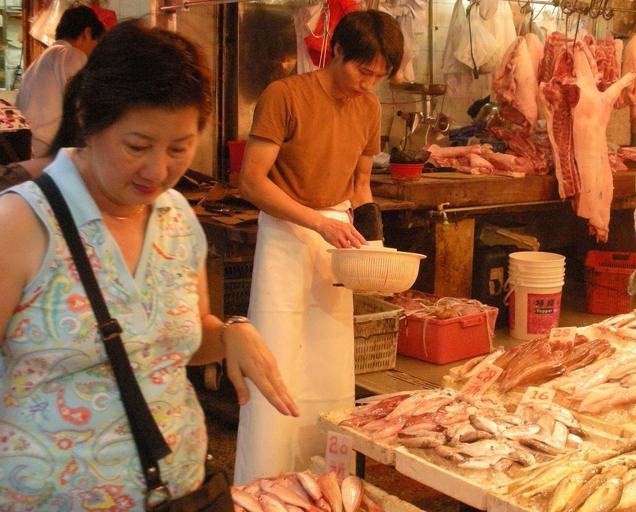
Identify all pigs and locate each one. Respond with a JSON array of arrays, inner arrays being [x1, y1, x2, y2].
[[539, 47, 636, 244]]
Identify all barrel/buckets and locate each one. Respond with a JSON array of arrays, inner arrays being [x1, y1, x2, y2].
[[226, 138, 248, 175], [503, 252, 567, 341]]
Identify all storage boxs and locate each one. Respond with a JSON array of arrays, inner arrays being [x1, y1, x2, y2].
[[582, 249, 636, 316], [393, 289, 499, 364]]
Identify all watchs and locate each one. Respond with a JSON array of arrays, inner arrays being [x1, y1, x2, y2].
[[219, 314, 250, 344]]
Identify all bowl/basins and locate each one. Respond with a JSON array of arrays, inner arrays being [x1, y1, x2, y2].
[[388, 162, 425, 181]]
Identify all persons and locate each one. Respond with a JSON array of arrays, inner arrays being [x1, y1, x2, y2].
[[16, 6, 107, 160], [233, 9, 405, 484], [0, 18, 301, 512]]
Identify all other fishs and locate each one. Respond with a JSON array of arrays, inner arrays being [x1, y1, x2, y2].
[[337, 308, 636, 512]]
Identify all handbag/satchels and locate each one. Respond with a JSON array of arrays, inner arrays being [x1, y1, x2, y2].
[[146, 463, 234, 512]]
